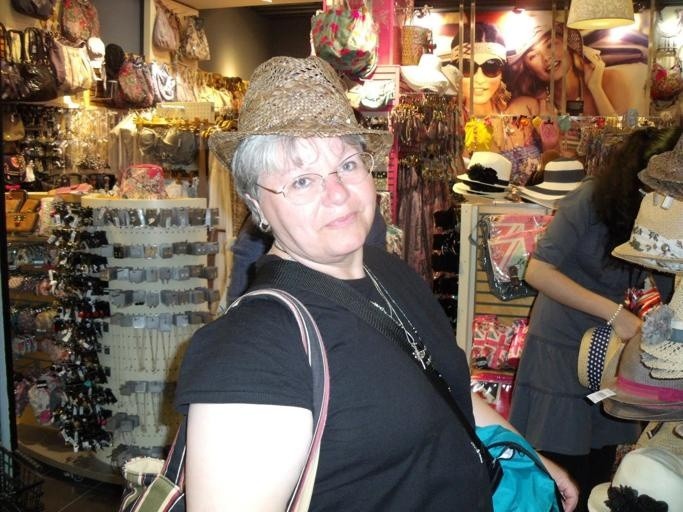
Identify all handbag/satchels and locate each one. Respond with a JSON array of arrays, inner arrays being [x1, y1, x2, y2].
[[118, 457, 187, 512], [11, 0, 56, 19], [474, 424, 565, 512], [181, 17, 211, 60], [312, 10, 378, 79], [649, 102, 680, 130], [480, 214, 555, 301], [0, 24, 95, 101], [470, 316, 529, 372], [106, 60, 154, 108], [157, 64, 195, 101], [154, 9, 183, 50], [61, 0, 100, 41], [652, 62, 683, 97]]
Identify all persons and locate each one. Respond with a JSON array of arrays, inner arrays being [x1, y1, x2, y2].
[[504, 130, 670, 511], [495, 8, 647, 122], [449, 19, 539, 156], [171, 54, 581, 512]]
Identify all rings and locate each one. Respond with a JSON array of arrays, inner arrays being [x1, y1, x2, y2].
[[592, 53, 602, 63]]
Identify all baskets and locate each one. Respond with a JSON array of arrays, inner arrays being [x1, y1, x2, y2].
[[401, 26, 427, 66]]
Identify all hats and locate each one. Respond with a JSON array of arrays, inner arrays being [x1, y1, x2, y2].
[[588, 448, 683, 512], [577, 281, 683, 422], [87, 37, 106, 68], [516, 158, 587, 210], [453, 151, 512, 199], [402, 54, 456, 97], [497, 12, 601, 69], [207, 56, 393, 169], [612, 133, 683, 274], [107, 44, 124, 76]]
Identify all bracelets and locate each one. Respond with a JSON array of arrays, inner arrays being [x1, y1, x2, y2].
[[605, 301, 626, 325]]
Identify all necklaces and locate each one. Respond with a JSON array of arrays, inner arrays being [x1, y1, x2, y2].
[[542, 76, 583, 118], [275, 240, 428, 374]]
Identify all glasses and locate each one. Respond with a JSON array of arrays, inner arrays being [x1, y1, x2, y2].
[[255, 151, 374, 205], [453, 59, 502, 78]]
